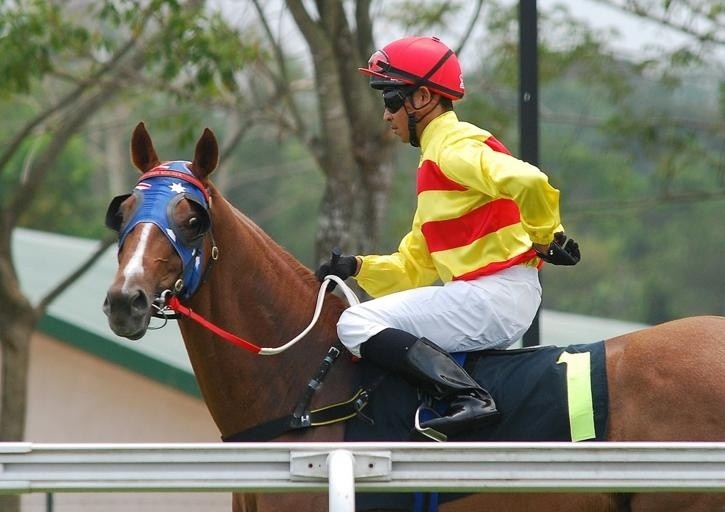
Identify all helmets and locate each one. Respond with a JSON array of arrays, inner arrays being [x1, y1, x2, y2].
[[357, 36, 465, 100]]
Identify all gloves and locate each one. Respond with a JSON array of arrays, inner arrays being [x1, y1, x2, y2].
[[315, 256, 357, 292], [532, 232, 580, 265]]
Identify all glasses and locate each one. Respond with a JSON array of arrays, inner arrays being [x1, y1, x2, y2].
[[382, 87, 405, 114]]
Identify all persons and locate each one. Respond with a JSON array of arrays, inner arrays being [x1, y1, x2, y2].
[[317, 37, 579, 430]]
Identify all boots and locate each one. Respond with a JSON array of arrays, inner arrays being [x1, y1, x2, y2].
[[394, 337, 501, 441]]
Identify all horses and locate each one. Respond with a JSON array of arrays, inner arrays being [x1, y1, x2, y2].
[[102, 120, 725, 512]]
